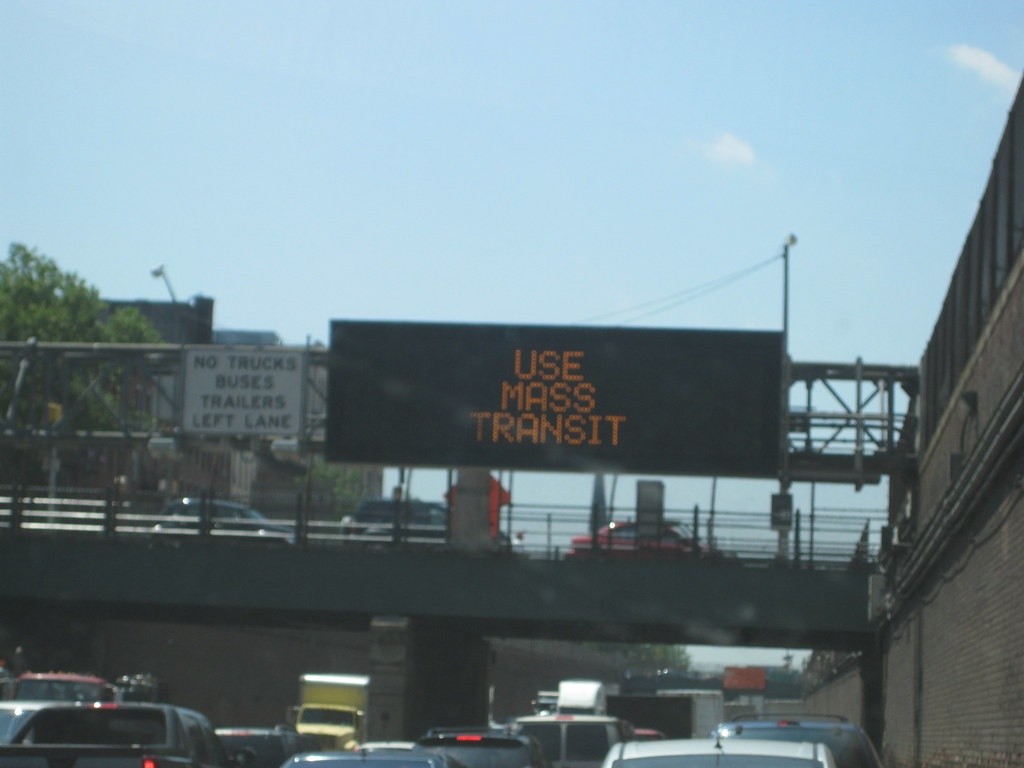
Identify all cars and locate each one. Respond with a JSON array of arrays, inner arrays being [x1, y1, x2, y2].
[[0, 662, 896, 768], [158, 493, 728, 560]]
